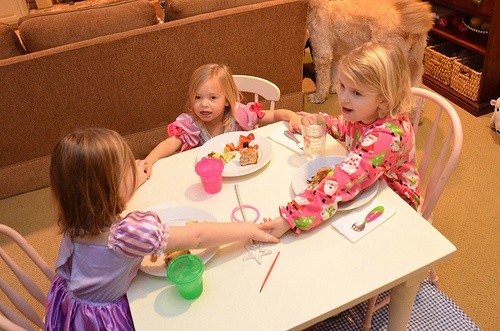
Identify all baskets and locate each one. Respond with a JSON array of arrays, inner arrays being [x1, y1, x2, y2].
[[424, 43, 465, 87], [451, 56, 482, 102]]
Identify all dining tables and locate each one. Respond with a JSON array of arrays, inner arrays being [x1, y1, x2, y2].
[[121, 120, 456, 331]]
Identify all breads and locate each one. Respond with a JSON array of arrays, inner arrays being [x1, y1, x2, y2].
[[165, 250, 191, 266], [239, 147, 258, 166], [211, 152, 236, 163]]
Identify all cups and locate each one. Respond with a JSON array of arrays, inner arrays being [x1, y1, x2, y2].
[[167, 253, 203, 299], [196, 157, 225, 194], [301, 112, 326, 156]]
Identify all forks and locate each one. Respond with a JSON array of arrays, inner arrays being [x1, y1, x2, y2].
[[351, 205, 384, 231]]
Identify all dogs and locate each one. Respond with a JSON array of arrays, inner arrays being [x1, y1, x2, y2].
[[308, 0, 433, 125]]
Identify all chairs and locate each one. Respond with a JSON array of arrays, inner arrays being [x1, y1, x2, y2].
[[403, 87, 463, 220], [0, 223, 57, 331], [232, 75, 280, 131]]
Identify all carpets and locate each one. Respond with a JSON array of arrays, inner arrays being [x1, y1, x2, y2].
[[310, 280, 477, 331]]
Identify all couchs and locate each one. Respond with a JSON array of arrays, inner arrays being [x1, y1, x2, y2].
[[0, 0, 307, 200]]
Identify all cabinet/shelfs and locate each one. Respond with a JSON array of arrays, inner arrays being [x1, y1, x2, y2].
[[422, 0, 500, 116]]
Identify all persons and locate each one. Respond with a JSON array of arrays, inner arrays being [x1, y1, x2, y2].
[[256, 42, 422, 238], [45, 128, 280, 331], [142, 63, 312, 179]]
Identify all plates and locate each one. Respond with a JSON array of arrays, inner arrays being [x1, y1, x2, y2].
[[138, 206, 219, 277], [195, 131, 272, 177], [292, 156, 379, 213]]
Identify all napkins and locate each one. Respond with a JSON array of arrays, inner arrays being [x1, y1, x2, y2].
[[267, 125, 306, 157], [332, 199, 396, 243]]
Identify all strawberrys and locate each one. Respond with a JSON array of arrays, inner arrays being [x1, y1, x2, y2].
[[226, 133, 255, 151]]
